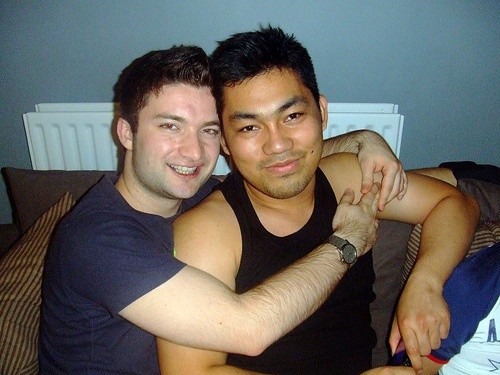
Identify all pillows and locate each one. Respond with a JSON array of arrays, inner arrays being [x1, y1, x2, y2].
[[0, 192, 76, 375], [398, 221, 500, 297]]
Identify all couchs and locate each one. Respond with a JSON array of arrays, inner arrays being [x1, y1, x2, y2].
[[0, 166, 499, 375]]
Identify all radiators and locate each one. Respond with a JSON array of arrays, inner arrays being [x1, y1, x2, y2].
[[21, 103, 404, 176]]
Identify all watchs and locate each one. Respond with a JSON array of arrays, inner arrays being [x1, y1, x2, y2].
[[324, 234, 358, 270]]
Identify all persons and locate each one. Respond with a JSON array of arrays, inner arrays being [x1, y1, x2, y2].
[[39, 44, 408, 375], [156, 22, 481, 375], [421, 241, 500, 375]]
